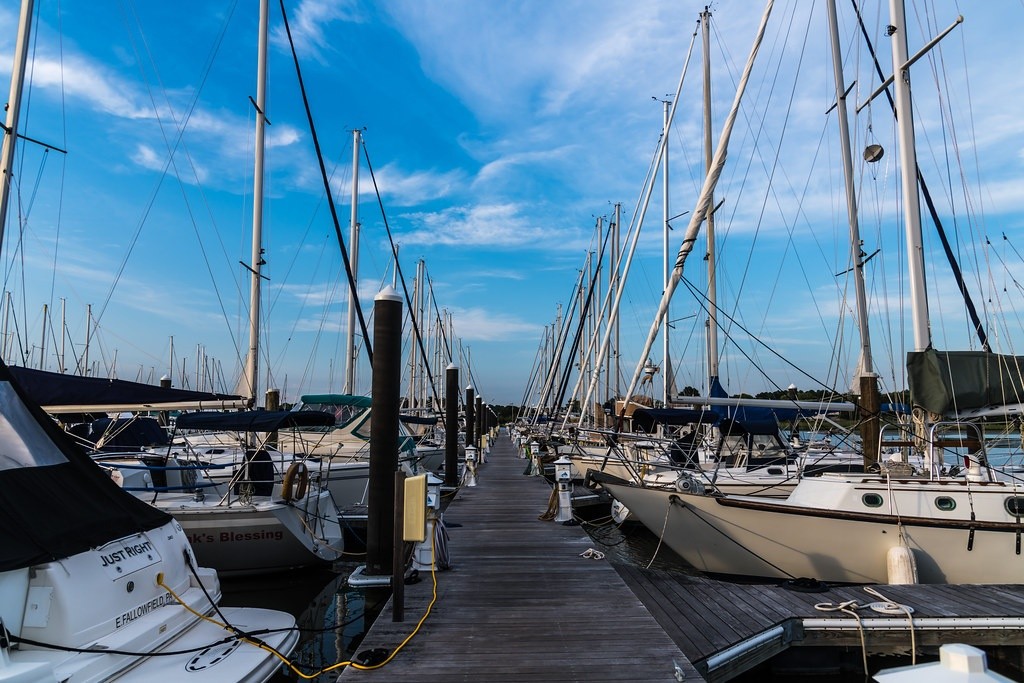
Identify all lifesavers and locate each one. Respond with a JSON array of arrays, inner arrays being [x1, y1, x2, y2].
[[283, 462, 308, 499]]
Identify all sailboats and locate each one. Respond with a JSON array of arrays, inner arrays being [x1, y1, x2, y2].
[[505, 0, 1023, 585], [0, 0, 503, 683]]
